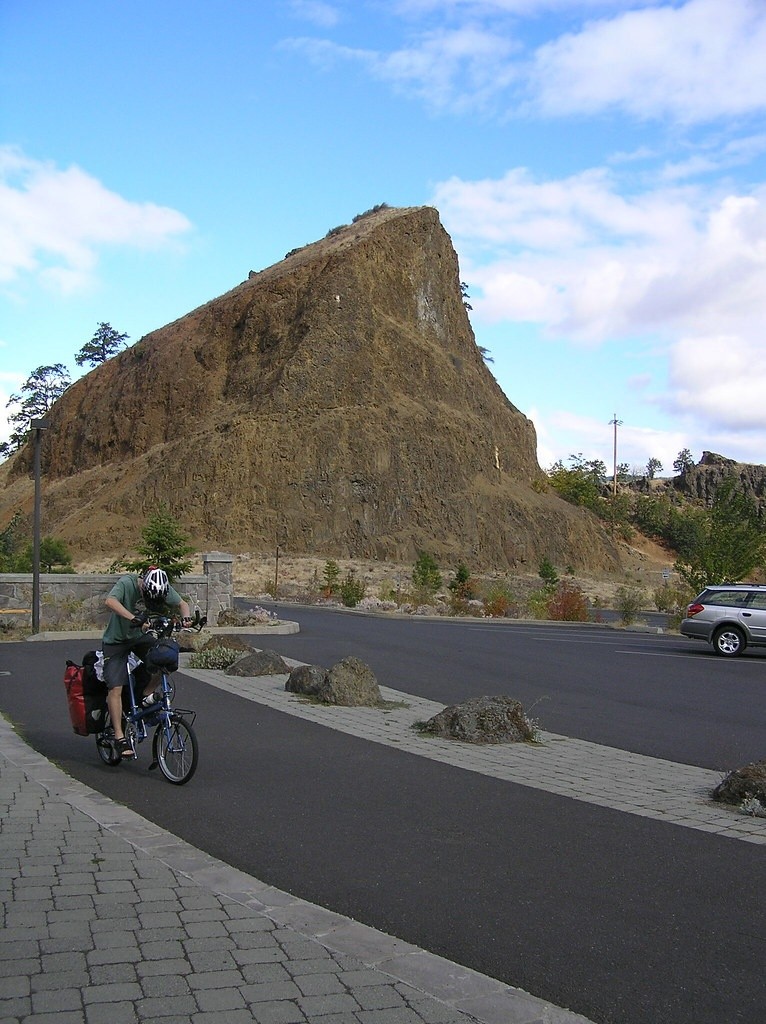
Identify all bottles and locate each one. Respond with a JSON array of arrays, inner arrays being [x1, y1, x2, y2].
[[141, 693, 159, 708]]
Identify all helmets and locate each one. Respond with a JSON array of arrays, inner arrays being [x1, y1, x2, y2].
[[143, 568, 169, 612]]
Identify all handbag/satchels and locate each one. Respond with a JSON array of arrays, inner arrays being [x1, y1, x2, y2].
[[64, 649, 105, 736], [149, 639, 179, 672]]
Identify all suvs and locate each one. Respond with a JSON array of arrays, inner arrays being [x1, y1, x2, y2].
[[681, 583, 766, 654]]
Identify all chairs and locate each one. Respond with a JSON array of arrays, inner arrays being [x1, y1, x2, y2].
[[735, 598, 743, 607]]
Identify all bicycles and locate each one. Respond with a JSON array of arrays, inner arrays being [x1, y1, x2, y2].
[[95, 610, 208, 786]]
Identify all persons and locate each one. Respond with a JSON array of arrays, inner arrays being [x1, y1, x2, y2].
[[102, 566, 193, 759]]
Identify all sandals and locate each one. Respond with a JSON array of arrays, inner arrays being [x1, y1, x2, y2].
[[114, 736, 134, 758], [140, 689, 159, 727]]
